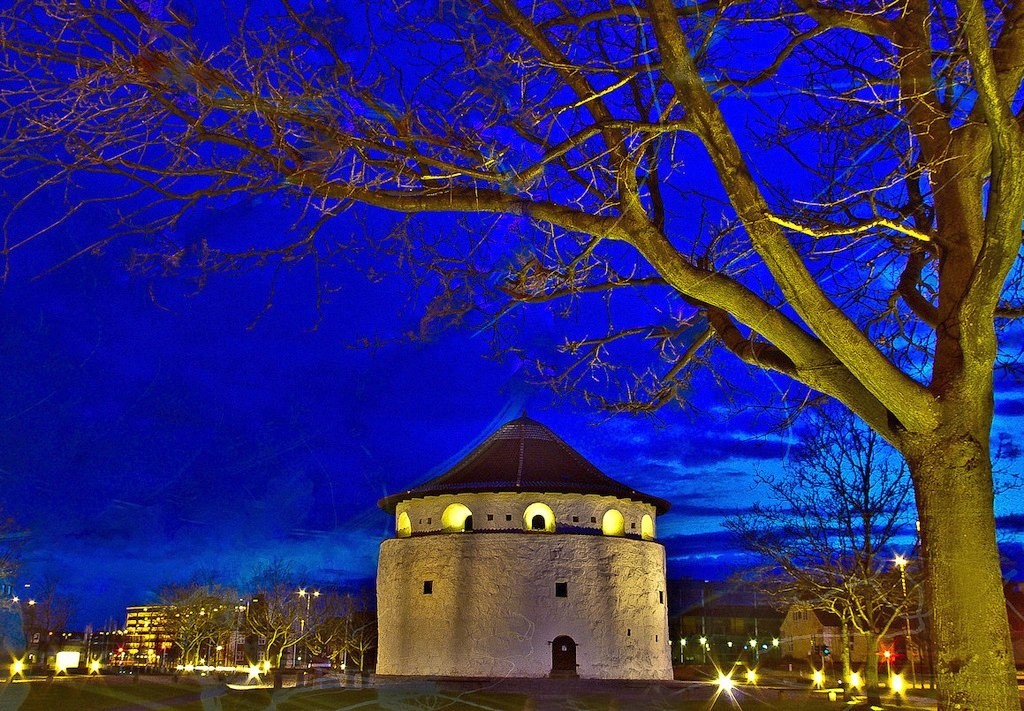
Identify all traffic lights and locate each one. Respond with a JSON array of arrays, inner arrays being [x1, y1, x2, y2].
[[825, 648, 830, 654]]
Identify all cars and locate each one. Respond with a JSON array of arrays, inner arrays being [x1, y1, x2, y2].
[[717, 662, 749, 685]]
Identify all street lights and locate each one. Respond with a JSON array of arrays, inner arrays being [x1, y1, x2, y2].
[[884, 649, 892, 690], [895, 556, 917, 689]]
